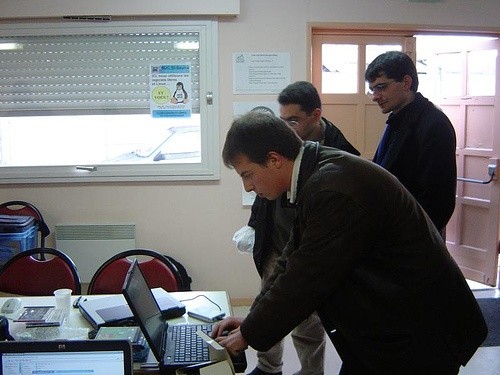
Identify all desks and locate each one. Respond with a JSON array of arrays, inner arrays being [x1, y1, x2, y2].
[[0, 289, 249, 375]]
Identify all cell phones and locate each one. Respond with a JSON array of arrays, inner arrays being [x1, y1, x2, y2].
[[189, 306, 225, 322]]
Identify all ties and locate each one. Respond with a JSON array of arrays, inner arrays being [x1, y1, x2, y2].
[[377, 122, 391, 163]]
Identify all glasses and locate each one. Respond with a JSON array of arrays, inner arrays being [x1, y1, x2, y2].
[[287, 112, 312, 129], [368, 75, 404, 95]]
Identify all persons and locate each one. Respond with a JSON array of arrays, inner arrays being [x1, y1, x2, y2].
[[365, 51, 457, 243], [247, 81, 360, 375], [208, 112, 493, 375]]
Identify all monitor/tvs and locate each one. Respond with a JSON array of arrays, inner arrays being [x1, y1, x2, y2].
[[0, 339, 134, 375]]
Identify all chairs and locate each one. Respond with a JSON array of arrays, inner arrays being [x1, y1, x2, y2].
[[87, 247, 186, 294], [0, 246, 82, 295], [0, 200, 48, 259]]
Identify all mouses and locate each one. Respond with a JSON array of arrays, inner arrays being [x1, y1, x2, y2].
[[1, 298, 22, 313], [72, 296, 88, 308]]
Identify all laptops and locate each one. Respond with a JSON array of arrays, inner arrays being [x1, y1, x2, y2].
[[122, 258, 247, 371], [80, 286, 185, 329]]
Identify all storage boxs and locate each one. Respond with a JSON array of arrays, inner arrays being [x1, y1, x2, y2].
[[0, 224, 38, 267]]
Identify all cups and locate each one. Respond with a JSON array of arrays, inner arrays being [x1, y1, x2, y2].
[[54, 289, 72, 316]]
